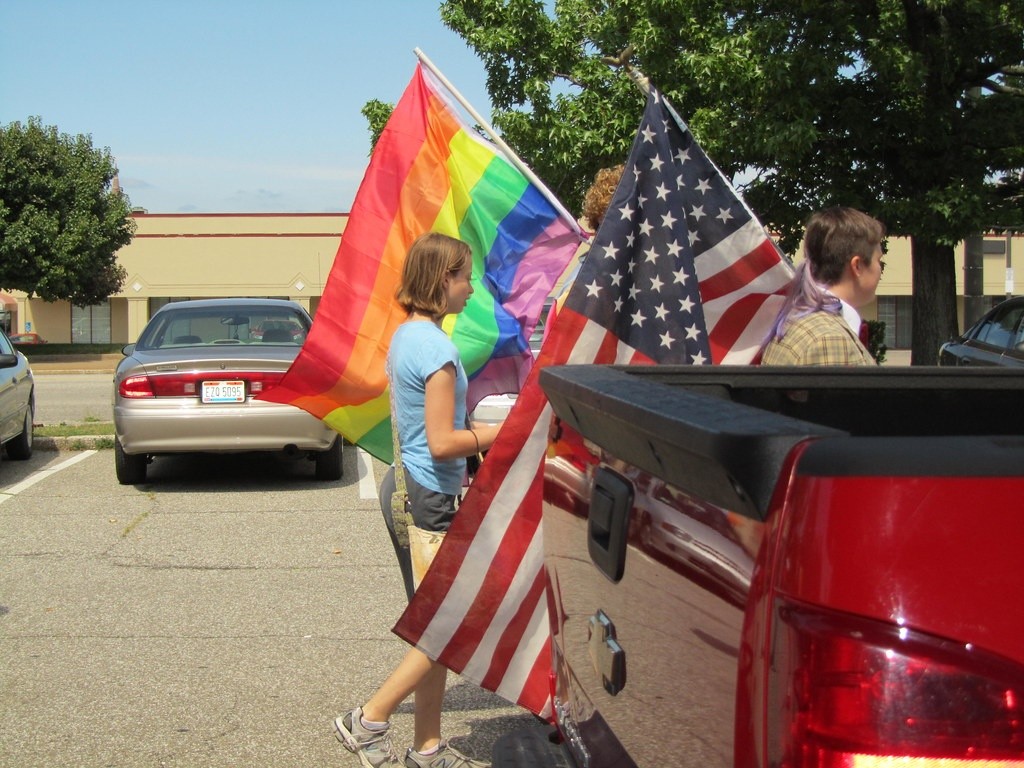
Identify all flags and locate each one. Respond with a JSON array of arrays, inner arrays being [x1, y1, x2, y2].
[[392, 81, 799, 723], [252, 63, 588, 487]]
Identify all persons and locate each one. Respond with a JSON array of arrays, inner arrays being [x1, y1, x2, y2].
[[583, 163, 625, 226], [329, 232, 503, 768], [764, 206, 884, 365]]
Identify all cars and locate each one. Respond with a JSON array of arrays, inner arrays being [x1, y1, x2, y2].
[[114, 298, 344, 486], [937, 297, 1024, 369], [0, 328, 35, 460]]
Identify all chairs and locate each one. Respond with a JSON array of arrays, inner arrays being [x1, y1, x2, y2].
[[261, 330, 294, 343], [172, 336, 202, 345]]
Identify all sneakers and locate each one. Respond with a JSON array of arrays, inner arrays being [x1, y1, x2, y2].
[[330, 705, 409, 768], [405, 737, 485, 768]]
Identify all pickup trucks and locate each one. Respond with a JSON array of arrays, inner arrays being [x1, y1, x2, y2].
[[535, 361, 1024, 768]]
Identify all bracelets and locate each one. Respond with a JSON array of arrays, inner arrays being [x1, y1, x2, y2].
[[470, 429, 480, 453]]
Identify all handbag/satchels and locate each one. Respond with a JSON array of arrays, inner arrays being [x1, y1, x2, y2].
[[407, 525, 449, 594]]
